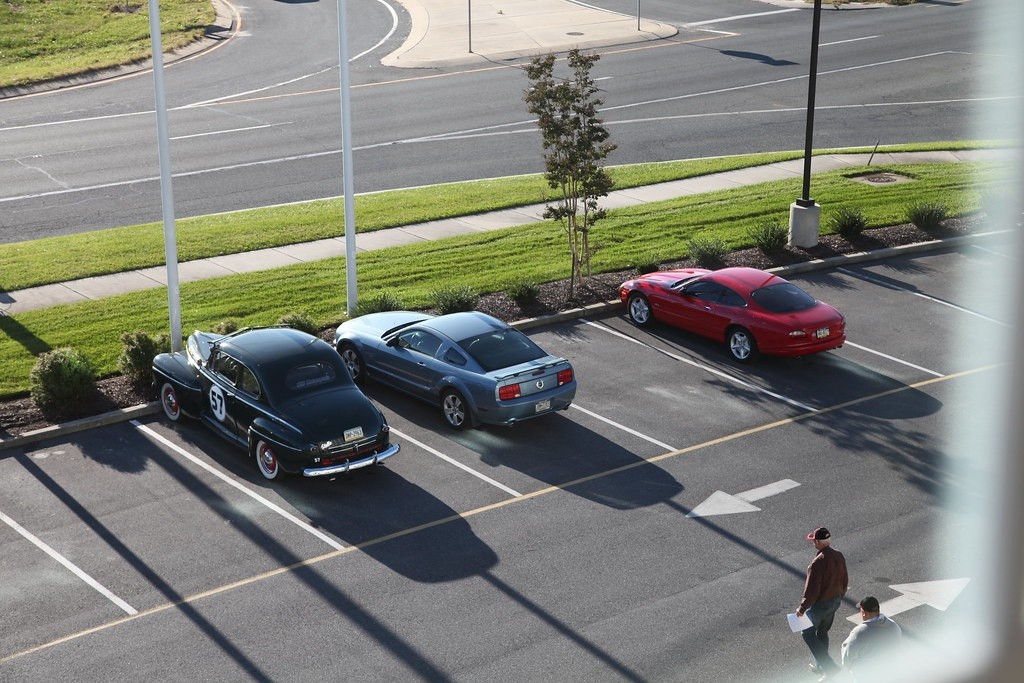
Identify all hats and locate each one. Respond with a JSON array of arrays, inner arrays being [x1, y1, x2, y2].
[[808, 527, 831, 540], [856, 597, 879, 612]]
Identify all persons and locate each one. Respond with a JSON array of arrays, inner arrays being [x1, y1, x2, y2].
[[795, 528, 849, 683], [839, 597, 902, 683]]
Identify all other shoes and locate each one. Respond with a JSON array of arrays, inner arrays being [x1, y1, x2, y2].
[[809, 663, 825, 674], [818, 662, 842, 683]]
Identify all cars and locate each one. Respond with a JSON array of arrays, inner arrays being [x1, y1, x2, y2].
[[332, 311, 577, 431], [152, 324, 402, 481], [617, 267, 846, 364]]
